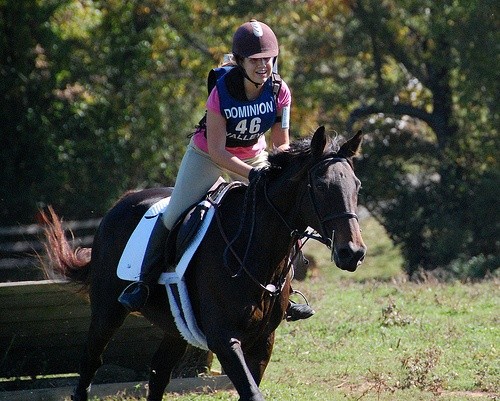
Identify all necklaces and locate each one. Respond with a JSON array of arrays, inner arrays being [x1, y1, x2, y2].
[[119, 18, 316, 322]]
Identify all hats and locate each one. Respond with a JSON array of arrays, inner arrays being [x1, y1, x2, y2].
[[232, 19, 279, 59]]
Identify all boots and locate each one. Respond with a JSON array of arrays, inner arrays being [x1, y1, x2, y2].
[[286, 298, 312, 319], [121, 213, 183, 311]]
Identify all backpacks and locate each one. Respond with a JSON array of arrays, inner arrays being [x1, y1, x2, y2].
[[198, 65, 282, 132]]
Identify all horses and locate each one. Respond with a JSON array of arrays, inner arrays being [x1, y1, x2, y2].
[[27, 125, 367, 401]]
